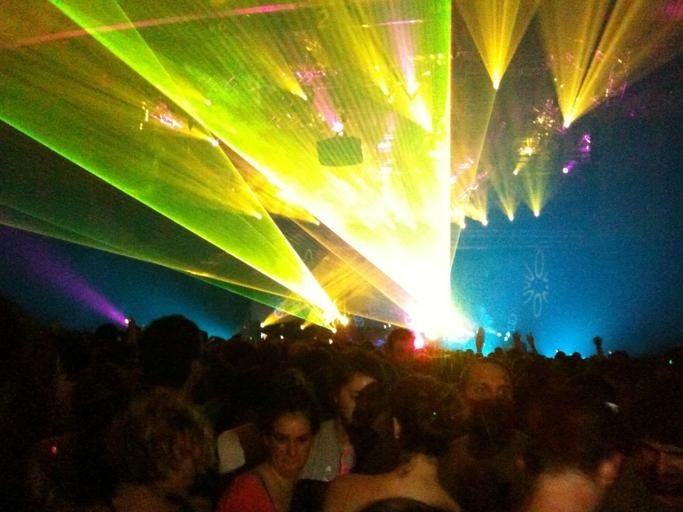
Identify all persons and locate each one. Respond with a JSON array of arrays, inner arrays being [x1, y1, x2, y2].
[[1, 311, 682, 511]]
